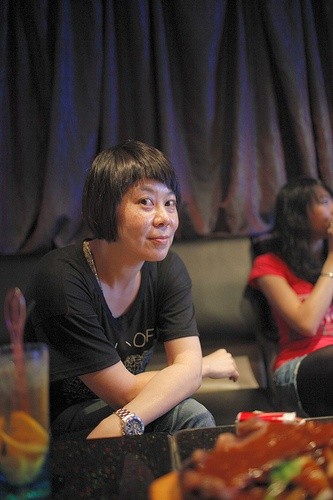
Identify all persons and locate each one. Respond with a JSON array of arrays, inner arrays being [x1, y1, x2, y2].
[[247, 176, 333, 417], [0, 139, 240, 440]]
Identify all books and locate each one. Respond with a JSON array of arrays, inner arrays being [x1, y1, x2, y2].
[[233, 410, 306, 425]]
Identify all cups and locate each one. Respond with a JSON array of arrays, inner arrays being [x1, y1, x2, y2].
[[0, 343, 52, 500]]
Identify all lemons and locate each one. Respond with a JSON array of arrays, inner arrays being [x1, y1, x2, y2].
[[0, 410, 51, 454]]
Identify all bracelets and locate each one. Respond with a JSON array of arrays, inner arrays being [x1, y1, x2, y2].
[[320, 272, 333, 278]]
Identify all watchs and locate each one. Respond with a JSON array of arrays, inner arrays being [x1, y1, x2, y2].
[[112, 408, 144, 436]]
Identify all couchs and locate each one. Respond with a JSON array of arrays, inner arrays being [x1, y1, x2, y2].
[[0, 236, 333, 441]]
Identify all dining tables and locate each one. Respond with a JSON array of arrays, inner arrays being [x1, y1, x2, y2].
[[0, 417, 333, 500]]
